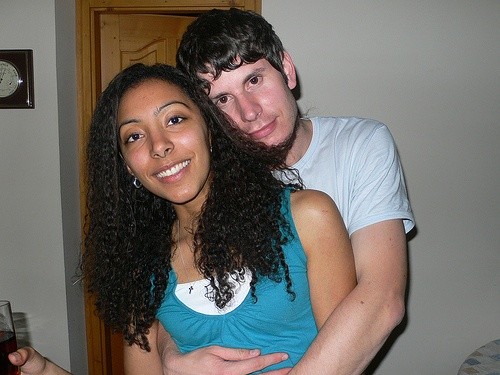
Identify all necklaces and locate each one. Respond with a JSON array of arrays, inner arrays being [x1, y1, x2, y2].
[[177, 220, 202, 294]]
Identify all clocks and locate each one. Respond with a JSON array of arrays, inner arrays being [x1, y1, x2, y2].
[[0, 49, 35, 111]]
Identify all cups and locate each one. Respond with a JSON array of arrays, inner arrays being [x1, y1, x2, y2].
[[0, 300, 19, 375]]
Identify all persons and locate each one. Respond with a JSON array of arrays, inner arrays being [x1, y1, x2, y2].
[[155, 7, 416, 375], [8, 64, 357, 375]]
